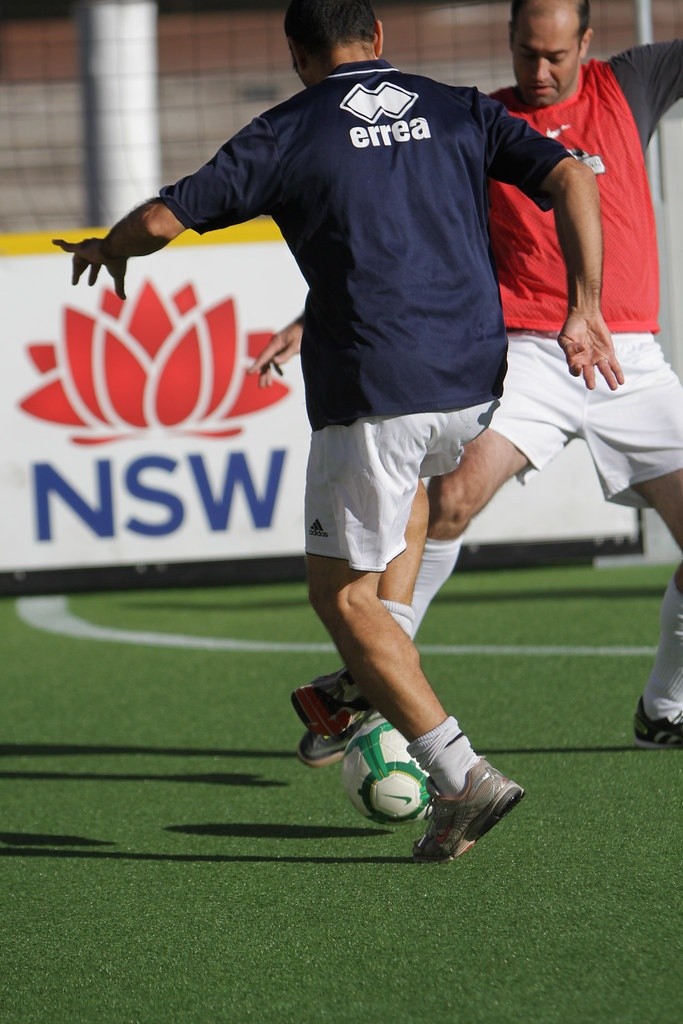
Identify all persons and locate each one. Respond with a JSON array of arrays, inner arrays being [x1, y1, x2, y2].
[[247, 0, 683, 765], [52, 0, 624, 863]]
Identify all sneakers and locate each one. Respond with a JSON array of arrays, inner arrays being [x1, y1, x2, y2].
[[634, 695, 683, 748], [291, 666, 372, 735], [297, 710, 376, 767], [413, 756, 525, 865]]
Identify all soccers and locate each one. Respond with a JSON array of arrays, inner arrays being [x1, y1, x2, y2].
[[341, 712, 433, 827]]
